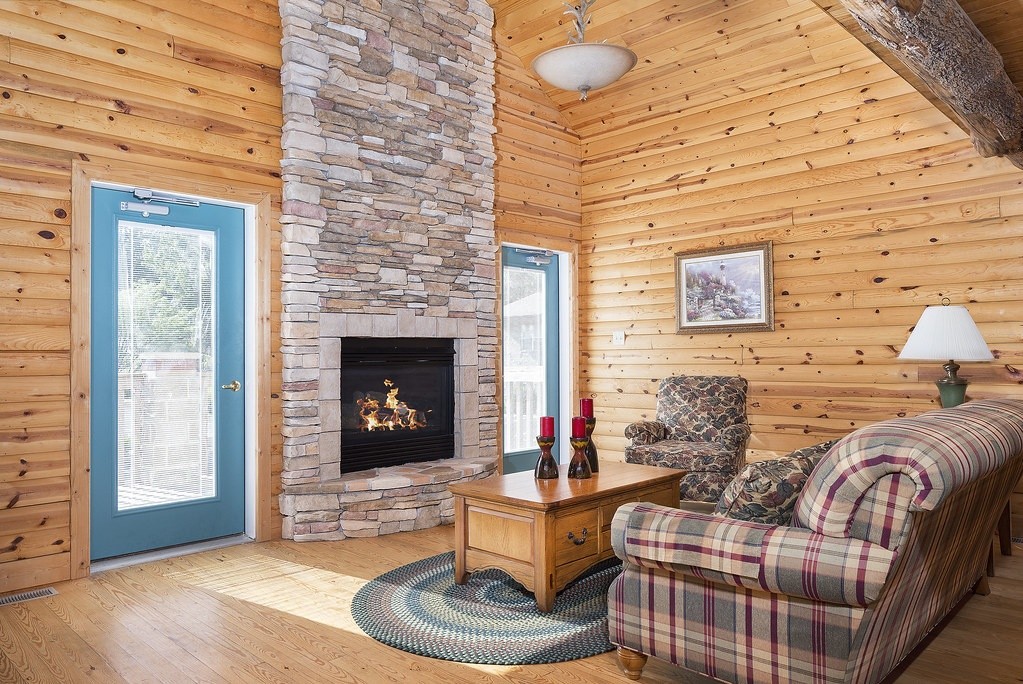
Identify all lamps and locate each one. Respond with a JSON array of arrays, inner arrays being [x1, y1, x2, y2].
[[531, 0, 638, 102], [896, 306, 994, 408]]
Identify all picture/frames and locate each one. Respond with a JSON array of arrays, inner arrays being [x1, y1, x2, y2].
[[673, 239, 775, 336]]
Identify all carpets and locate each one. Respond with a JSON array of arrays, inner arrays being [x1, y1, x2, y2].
[[351, 549, 617, 665]]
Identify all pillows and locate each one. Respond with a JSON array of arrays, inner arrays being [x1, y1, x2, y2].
[[711, 438, 839, 524]]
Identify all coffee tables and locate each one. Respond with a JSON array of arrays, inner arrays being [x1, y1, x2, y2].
[[448, 460, 687, 613]]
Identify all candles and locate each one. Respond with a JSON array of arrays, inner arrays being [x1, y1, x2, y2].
[[572, 416, 586, 438], [580, 398, 593, 418], [540, 415, 555, 437]]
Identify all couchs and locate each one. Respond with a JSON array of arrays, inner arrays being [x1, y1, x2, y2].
[[623, 374, 752, 511], [607, 396, 1023, 684]]
